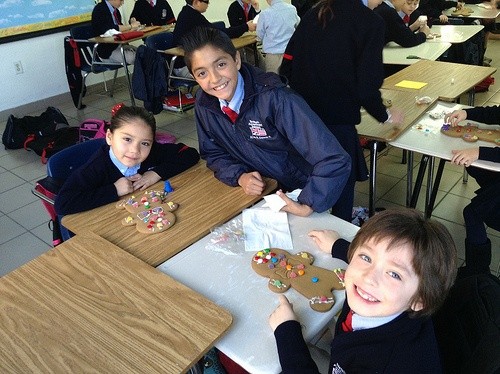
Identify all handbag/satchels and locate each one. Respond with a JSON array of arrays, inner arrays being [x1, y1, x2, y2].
[[2, 106, 70, 150], [78, 118, 111, 143], [24, 126, 80, 164]]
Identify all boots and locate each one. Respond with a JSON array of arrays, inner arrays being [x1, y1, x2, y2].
[[465, 237, 491, 276]]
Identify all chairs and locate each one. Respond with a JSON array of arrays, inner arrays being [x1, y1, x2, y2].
[[66, 23, 136, 108], [146, 32, 193, 113], [213, 22, 246, 63]]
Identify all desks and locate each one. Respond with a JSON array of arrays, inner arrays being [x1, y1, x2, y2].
[[0, 0, 500, 374]]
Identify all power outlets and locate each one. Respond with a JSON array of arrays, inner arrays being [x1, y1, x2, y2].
[[14, 60, 23, 74]]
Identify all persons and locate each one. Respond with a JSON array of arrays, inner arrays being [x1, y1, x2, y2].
[[278, 0, 404, 223], [256, 0, 301, 74], [55, 103, 200, 216], [129, 0, 177, 44], [268, 207, 458, 374], [170, 0, 262, 79], [91, 0, 138, 65], [445, 104, 500, 275], [227, 0, 262, 27], [291, 0, 465, 48], [184, 27, 352, 217]]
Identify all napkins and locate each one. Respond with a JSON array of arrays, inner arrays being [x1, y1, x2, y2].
[[263, 189, 303, 212], [243, 207, 294, 252]]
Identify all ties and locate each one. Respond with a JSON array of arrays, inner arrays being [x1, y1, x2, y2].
[[244, 2, 248, 22], [402, 14, 410, 22], [342, 309, 352, 332], [222, 106, 238, 123], [150, 0, 154, 7], [114, 8, 120, 25]]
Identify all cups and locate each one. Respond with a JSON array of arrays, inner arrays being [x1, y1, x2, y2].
[[419, 15, 427, 28]]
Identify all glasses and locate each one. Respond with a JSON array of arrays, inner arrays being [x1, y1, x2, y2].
[[198, 0, 208, 4]]
